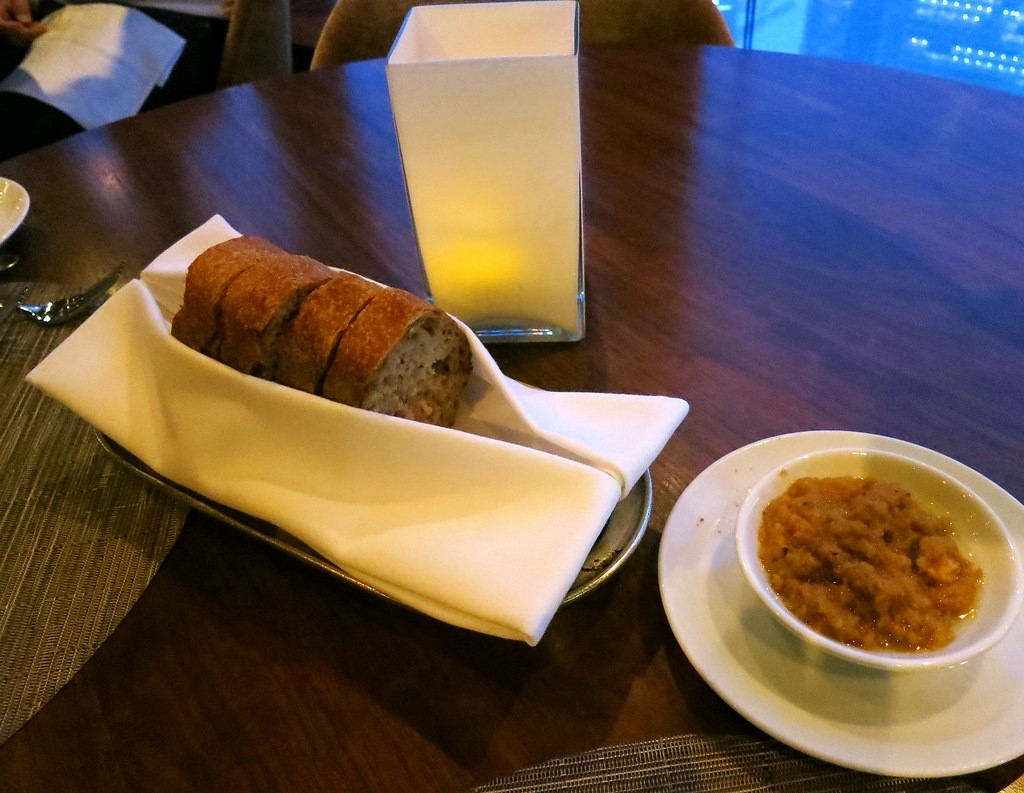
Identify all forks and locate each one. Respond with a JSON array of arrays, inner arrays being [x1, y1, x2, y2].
[[0, 260, 126, 326]]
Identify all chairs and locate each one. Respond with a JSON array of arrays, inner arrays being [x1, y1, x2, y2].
[[310, 0, 734, 71], [216, 0, 292, 89]]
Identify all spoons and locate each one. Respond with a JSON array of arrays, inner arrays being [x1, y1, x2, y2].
[[0, 252, 19, 271]]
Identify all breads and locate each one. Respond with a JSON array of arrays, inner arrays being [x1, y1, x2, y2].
[[170, 233, 288, 355], [321, 285, 474, 428], [273, 270, 386, 395], [206, 253, 336, 379]]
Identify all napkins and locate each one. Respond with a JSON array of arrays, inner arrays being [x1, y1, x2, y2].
[[16, 213, 690, 648], [0, 3, 188, 130]]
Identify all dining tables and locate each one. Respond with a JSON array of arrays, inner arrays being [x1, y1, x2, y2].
[[0, 48, 1024, 793]]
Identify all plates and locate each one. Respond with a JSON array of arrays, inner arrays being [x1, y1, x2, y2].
[[656, 430, 1024, 793], [92, 379, 653, 605]]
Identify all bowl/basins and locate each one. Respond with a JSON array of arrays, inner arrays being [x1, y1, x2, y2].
[[736, 447, 1024, 674], [0, 177, 30, 246]]
